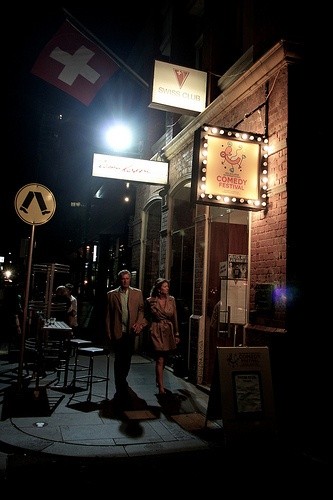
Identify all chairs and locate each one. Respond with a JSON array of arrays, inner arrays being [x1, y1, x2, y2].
[[14, 314, 40, 376]]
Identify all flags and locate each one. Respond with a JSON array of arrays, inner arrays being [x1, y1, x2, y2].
[[30, 19, 121, 106]]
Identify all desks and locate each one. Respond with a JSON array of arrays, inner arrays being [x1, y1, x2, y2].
[[36, 320, 73, 388]]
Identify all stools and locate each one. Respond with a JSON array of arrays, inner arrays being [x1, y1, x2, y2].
[[64, 338, 110, 402]]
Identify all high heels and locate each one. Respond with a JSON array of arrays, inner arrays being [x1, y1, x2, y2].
[[159, 388, 166, 395], [156, 379, 160, 387]]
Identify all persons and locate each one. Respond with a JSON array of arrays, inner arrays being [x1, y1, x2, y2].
[[53, 282, 78, 339], [104, 269, 144, 398], [132, 277, 180, 396]]
